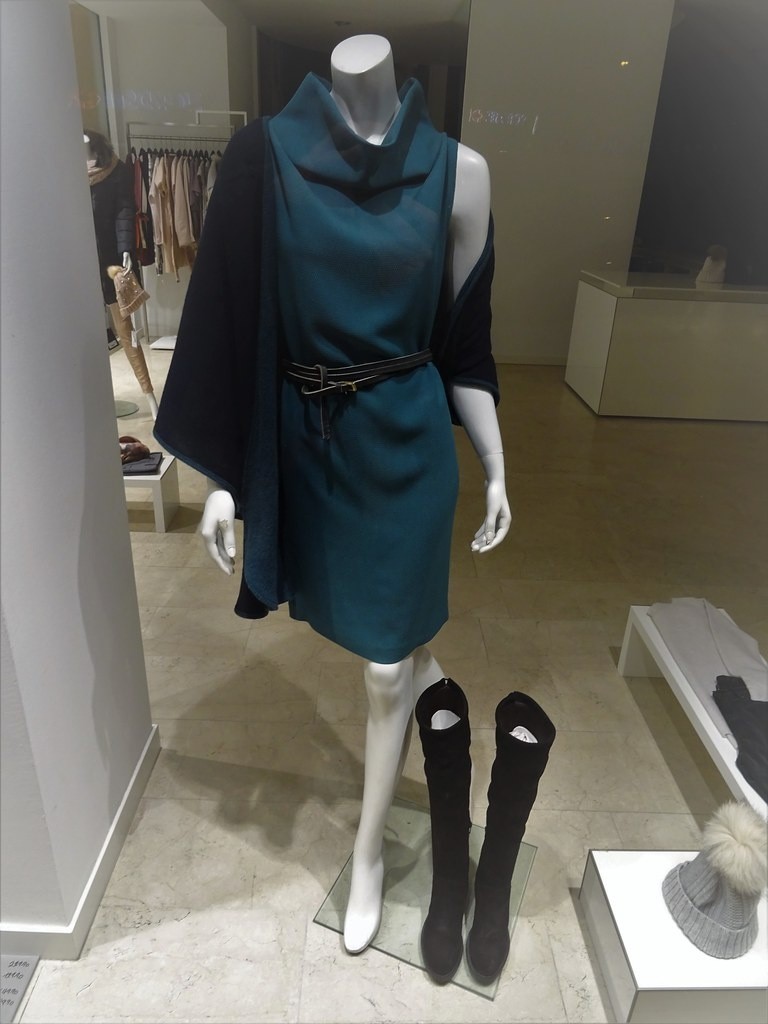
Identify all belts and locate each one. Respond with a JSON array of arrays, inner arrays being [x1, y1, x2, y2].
[[282, 347, 434, 441]]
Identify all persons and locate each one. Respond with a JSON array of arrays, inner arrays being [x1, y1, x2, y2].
[[82, 130, 157, 423], [154, 35, 511, 956]]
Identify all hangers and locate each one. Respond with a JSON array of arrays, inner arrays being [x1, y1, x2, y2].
[[130, 134, 229, 161]]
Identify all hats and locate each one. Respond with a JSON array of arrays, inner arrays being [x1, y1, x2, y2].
[[106, 264, 151, 318], [659, 797, 767, 960]]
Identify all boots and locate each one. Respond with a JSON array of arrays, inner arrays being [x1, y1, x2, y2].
[[412, 675, 474, 985], [465, 692, 557, 983]]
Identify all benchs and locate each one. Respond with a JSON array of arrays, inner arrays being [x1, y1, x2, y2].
[[123, 453, 181, 533], [618, 606, 768, 825]]
